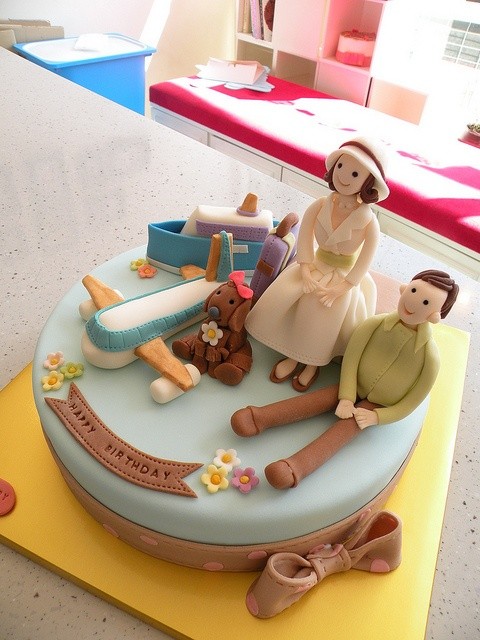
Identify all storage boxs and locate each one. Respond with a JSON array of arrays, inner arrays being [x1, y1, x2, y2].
[[13, 31, 156, 116]]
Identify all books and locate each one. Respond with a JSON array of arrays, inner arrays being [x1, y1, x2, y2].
[[243, 0, 273, 42]]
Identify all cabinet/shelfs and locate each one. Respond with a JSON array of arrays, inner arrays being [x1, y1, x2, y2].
[[231, 0, 444, 128], [149, 72, 480, 280]]
